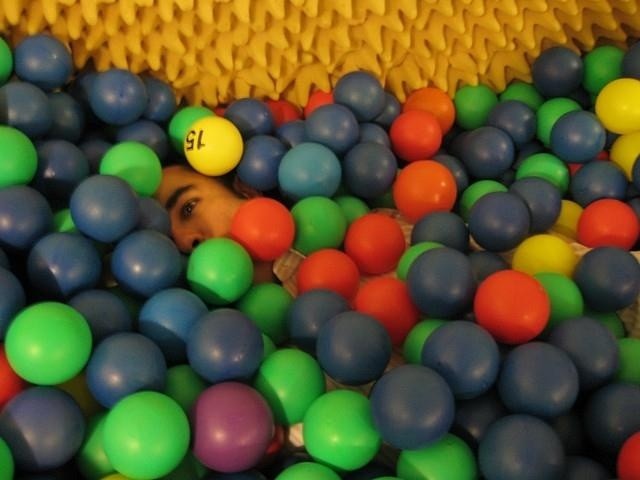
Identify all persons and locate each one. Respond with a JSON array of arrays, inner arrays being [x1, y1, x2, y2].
[[152, 152, 639, 472]]
